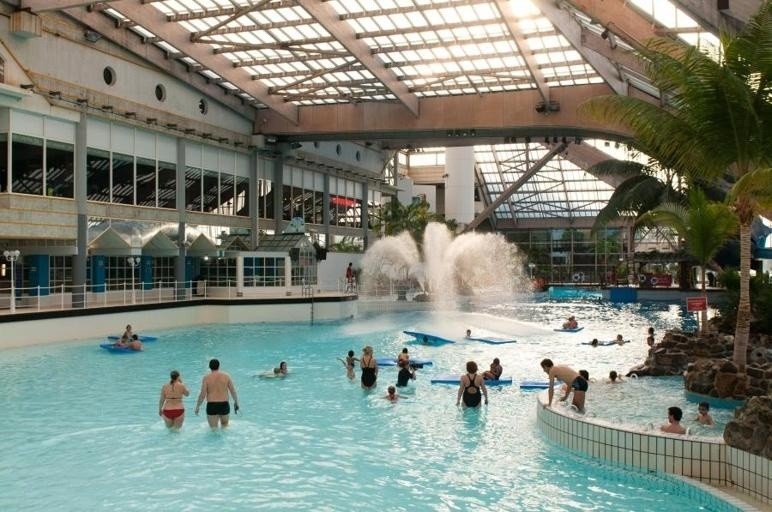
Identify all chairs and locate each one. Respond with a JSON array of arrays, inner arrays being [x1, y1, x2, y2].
[[302, 279, 313, 297]]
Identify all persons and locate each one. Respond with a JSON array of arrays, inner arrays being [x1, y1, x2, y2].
[[561, 315, 579, 330], [659, 406, 691, 435], [540, 358, 588, 414], [591, 338, 598, 345], [646, 336, 654, 346], [123, 324, 133, 338], [647, 326, 657, 337], [345, 262, 354, 294], [116, 336, 129, 346], [130, 334, 142, 351], [382, 386, 408, 402], [195, 358, 240, 428], [274, 360, 288, 374], [336, 345, 417, 388], [605, 370, 625, 384], [158, 370, 190, 429], [614, 334, 624, 342], [481, 357, 503, 381], [693, 400, 716, 427], [466, 330, 471, 336], [562, 369, 593, 391], [454, 360, 489, 409]]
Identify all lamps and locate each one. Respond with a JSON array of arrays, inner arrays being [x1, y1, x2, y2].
[[447, 130, 475, 137], [503, 135, 582, 144]]
